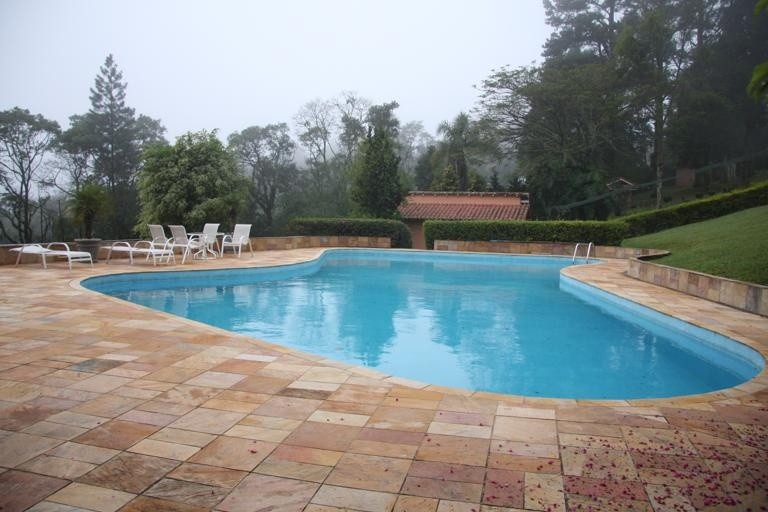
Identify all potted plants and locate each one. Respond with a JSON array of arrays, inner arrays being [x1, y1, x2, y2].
[[68, 179, 112, 264]]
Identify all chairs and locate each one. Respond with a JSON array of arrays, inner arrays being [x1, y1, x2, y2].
[[147, 223, 254, 265], [10, 242, 93, 268], [100, 240, 176, 266]]
